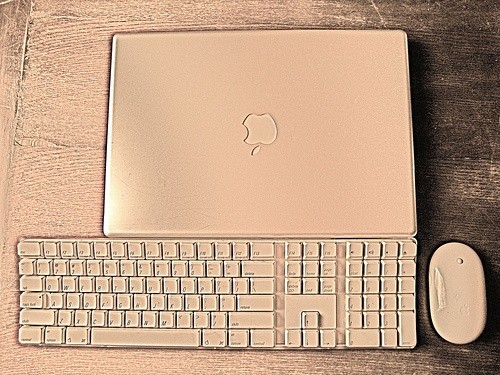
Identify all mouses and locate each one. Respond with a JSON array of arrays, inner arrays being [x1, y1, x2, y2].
[[428, 242, 487, 345]]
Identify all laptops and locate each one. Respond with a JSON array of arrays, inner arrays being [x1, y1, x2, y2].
[[104, 31, 417, 237]]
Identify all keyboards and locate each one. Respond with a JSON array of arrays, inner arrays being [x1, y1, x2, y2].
[[17, 238, 416, 351]]
